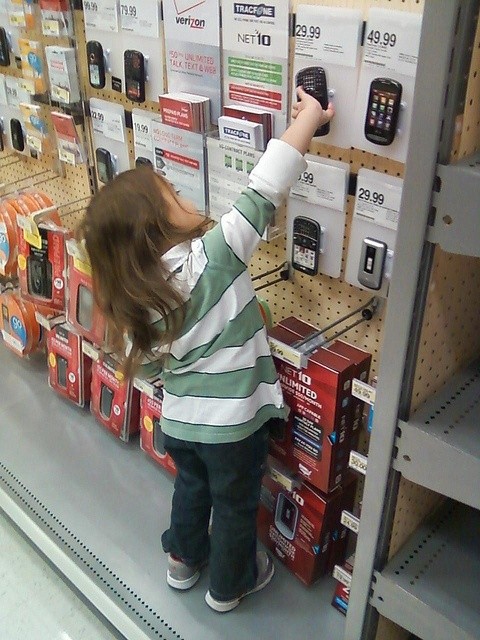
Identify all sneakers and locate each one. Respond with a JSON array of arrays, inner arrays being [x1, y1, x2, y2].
[[205, 550, 275, 613], [166, 523, 213, 591]]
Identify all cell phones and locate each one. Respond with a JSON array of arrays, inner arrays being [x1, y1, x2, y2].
[[124, 50, 145, 102], [292, 216, 320, 276], [358, 237, 387, 290], [57, 357, 67, 387], [100, 386, 114, 416], [365, 78, 402, 146], [295, 67, 330, 137], [96, 148, 113, 185], [0, 27, 11, 67], [280, 497, 297, 532], [154, 422, 166, 455], [135, 157, 153, 170], [11, 118, 24, 151], [86, 40, 105, 89]]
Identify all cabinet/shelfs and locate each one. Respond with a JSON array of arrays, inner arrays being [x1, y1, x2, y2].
[[340, 0, 478, 640]]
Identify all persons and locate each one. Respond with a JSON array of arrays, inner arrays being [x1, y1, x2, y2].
[[75, 84, 335, 614]]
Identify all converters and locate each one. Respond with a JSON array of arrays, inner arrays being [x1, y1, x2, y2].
[[30, 260, 46, 295]]
